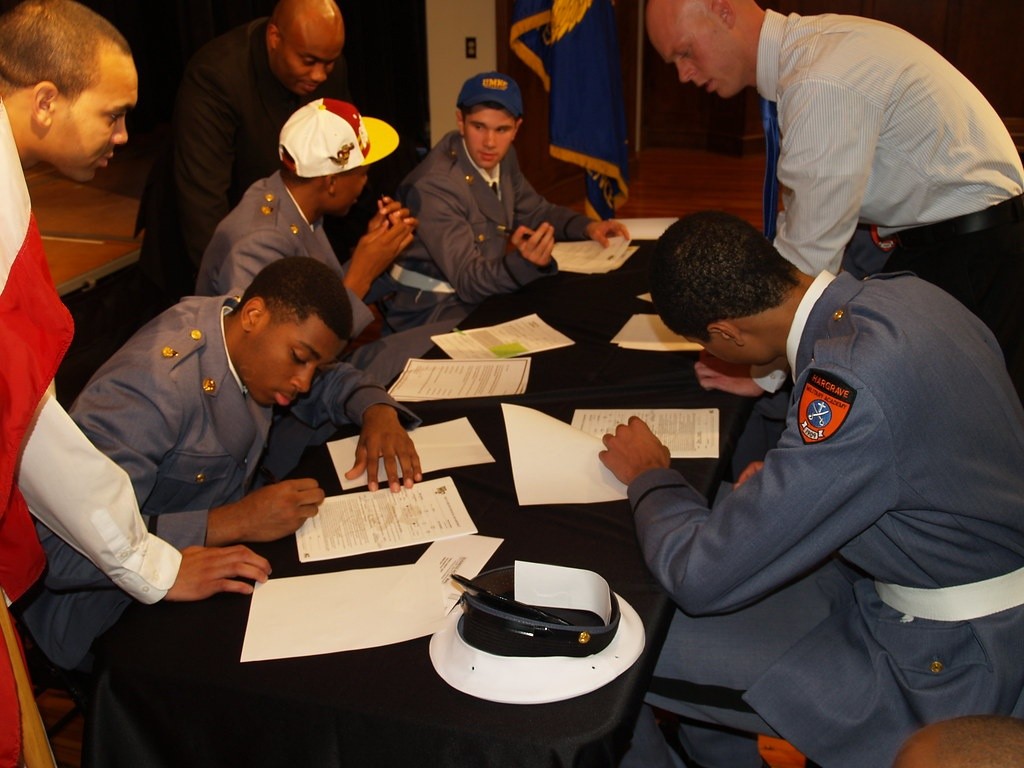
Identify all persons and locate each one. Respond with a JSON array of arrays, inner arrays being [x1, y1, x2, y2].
[[1, 0, 273, 601], [194, 98, 460, 377], [596, 210, 1023, 767], [132, 1, 418, 325], [373, 71, 632, 334], [646, 0, 1024, 403], [12, 259, 426, 705]]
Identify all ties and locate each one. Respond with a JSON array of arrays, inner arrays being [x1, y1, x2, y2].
[[760, 99, 779, 243]]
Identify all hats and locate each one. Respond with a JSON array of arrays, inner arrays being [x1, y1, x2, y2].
[[457, 72, 523, 123], [279, 98, 399, 178]]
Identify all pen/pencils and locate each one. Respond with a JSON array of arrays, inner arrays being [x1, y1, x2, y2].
[[376, 189, 393, 230], [496, 225, 532, 241], [258, 465, 279, 484]]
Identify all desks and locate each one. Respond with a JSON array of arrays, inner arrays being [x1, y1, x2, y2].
[[74, 237, 765, 767]]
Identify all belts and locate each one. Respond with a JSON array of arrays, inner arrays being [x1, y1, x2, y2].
[[875, 566, 1024, 621]]
